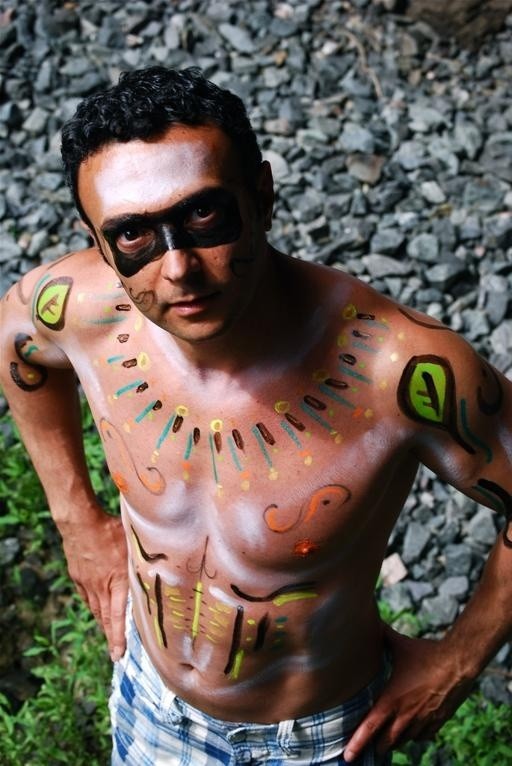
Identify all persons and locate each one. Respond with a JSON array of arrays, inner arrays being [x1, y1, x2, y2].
[[2, 66, 509, 766]]
